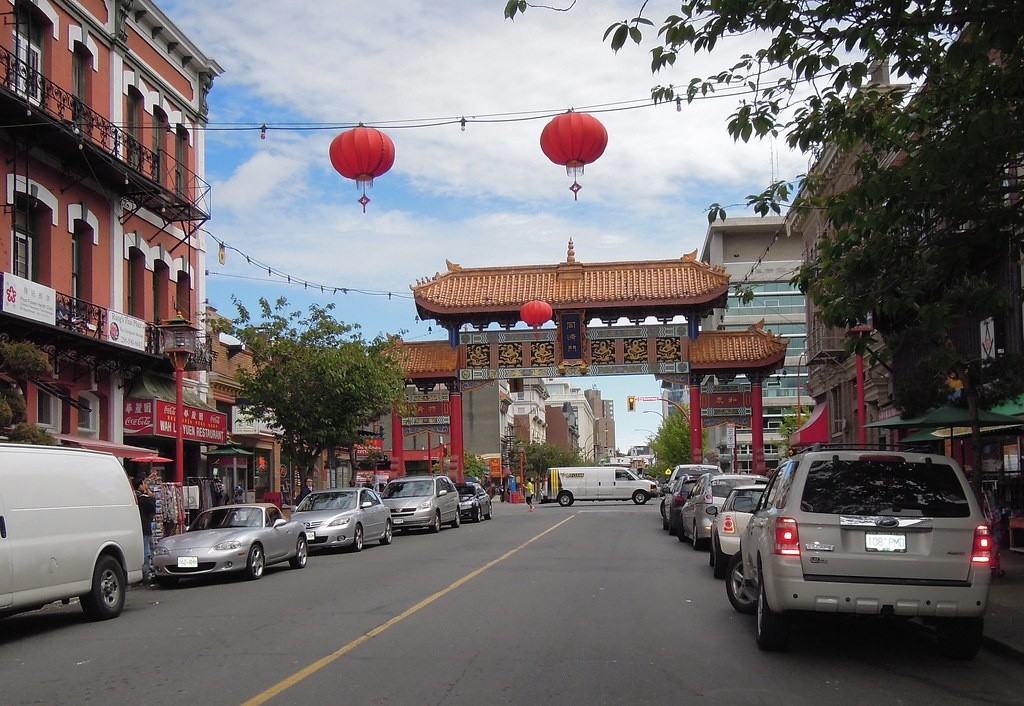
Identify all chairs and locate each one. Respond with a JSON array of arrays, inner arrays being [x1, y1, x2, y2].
[[56, 302, 88, 334]]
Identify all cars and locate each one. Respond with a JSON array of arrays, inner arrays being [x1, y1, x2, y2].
[[662, 474, 702, 538], [288, 487, 393, 552], [676, 474, 770, 552], [705, 484, 766, 580], [452, 481, 493, 522], [151, 502, 310, 588]]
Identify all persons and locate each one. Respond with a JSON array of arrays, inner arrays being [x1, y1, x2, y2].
[[300, 478, 313, 500], [522, 478, 536, 512], [131, 474, 156, 582], [363, 478, 374, 490]]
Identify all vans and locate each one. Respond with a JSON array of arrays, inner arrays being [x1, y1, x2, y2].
[[0, 440, 147, 623], [659, 465, 722, 529], [540, 468, 662, 504]]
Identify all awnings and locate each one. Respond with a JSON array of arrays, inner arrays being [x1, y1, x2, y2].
[[49, 432, 158, 458], [123, 373, 228, 445], [788, 401, 828, 447]]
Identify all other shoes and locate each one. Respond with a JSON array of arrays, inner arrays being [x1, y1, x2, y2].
[[530, 507, 535, 511]]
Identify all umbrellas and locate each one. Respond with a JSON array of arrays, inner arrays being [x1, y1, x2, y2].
[[130, 456, 173, 472], [861, 401, 1024, 469]]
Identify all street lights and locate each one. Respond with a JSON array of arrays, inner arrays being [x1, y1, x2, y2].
[[517, 442, 527, 504], [155, 310, 202, 531], [584, 429, 608, 462]]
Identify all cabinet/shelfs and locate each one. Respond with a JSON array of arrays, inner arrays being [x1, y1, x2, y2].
[[1009, 516, 1024, 553]]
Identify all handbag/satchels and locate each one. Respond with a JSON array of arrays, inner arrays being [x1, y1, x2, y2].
[[525, 488, 531, 497]]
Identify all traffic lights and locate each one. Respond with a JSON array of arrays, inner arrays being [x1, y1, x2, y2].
[[627, 395, 635, 412], [443, 445, 447, 456]]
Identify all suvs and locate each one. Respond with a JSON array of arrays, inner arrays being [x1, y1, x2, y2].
[[725, 441, 993, 661], [380, 474, 460, 534]]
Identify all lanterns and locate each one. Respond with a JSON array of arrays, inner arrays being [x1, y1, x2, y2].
[[330, 126, 395, 214], [540, 112, 609, 202], [520, 300, 552, 326]]
